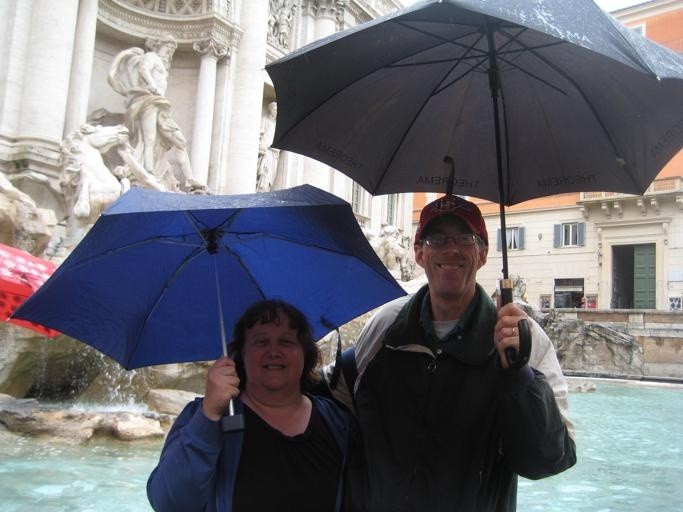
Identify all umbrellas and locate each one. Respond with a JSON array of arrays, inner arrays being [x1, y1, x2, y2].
[[265, 0, 683, 371], [7, 184, 407, 433], [0, 244, 64, 338]]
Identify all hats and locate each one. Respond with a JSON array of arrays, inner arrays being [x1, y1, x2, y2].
[[415, 195, 488, 246]]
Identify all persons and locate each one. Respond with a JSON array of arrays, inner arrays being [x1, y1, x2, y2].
[[276, 0, 294, 49], [147, 299, 370, 511], [137, 34, 205, 189], [256, 102, 282, 193], [312, 194, 577, 512]]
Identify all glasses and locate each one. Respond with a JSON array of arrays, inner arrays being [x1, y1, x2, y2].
[[425, 232, 483, 248]]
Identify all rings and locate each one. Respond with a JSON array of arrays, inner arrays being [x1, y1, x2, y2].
[[511, 327, 515, 336]]
